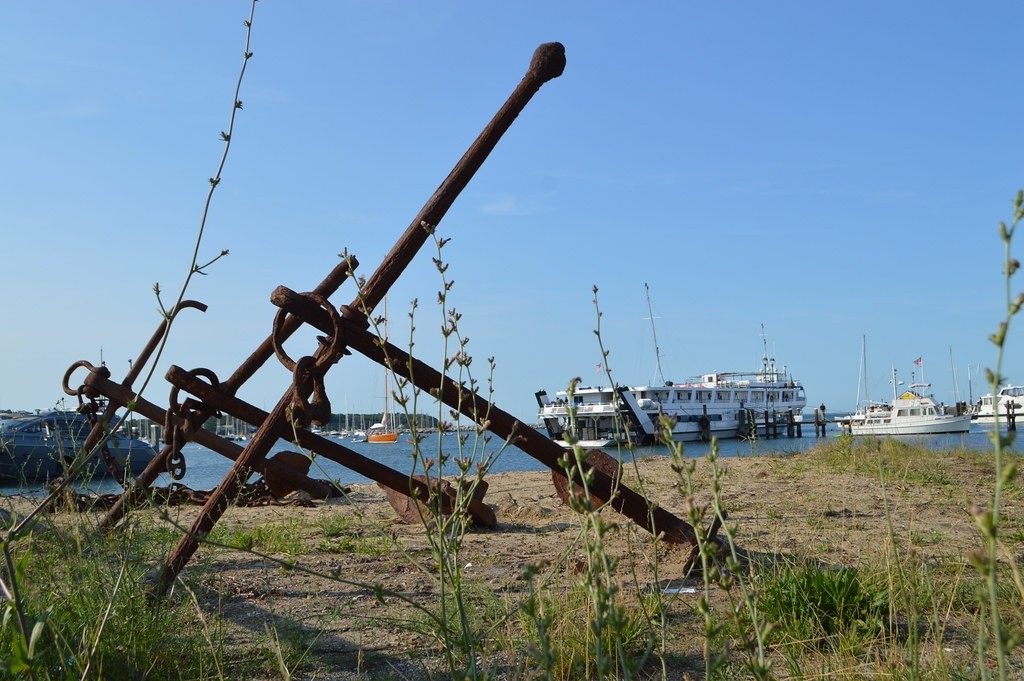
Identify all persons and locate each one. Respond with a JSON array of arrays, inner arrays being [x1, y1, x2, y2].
[[550, 396, 582, 405]]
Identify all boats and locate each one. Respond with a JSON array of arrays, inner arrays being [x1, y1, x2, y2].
[[969, 383, 1024, 425], [535, 321, 808, 450], [834, 336, 976, 435], [0, 407, 156, 481]]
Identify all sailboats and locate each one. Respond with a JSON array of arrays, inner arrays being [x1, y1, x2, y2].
[[366, 297, 399, 444]]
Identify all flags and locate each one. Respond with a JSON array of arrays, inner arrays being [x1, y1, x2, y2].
[[914, 356, 921, 367]]
